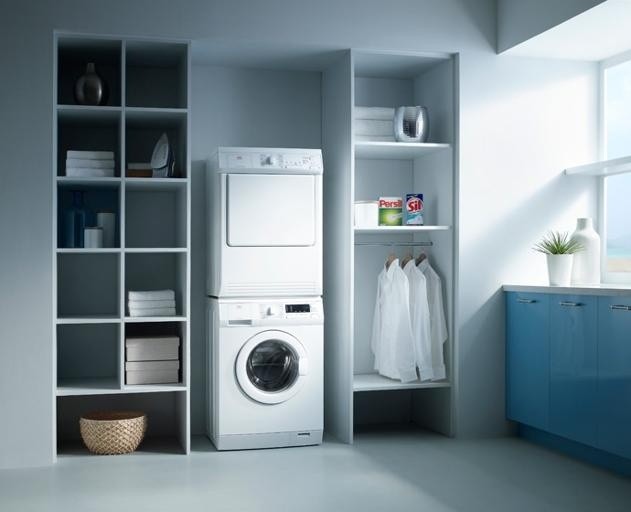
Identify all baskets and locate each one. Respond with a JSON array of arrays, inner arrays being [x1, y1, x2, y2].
[[78, 411, 149, 455]]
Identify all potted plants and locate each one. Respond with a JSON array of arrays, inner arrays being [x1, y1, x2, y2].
[[529, 227, 586, 287]]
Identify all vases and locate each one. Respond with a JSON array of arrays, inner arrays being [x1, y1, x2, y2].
[[571, 218, 601, 287]]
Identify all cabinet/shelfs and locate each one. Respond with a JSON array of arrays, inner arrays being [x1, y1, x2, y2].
[[599, 289, 627, 479], [321, 46, 461, 446], [52, 28, 191, 464], [546, 286, 600, 468], [500, 284, 547, 448]]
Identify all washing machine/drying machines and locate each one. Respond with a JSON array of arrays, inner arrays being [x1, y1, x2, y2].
[[203, 296, 325, 452], [203, 147, 324, 299]]
